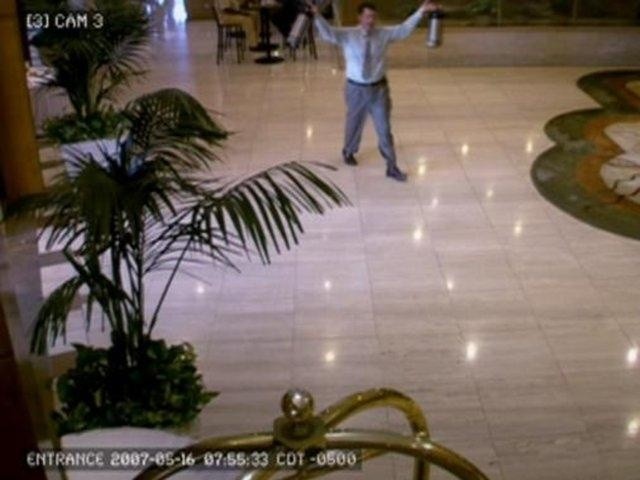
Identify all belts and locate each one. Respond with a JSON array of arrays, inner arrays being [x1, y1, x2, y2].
[[346, 75, 386, 86]]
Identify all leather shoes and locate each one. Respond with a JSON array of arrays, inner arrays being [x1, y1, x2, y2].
[[387, 168, 406, 180], [342, 148, 357, 166]]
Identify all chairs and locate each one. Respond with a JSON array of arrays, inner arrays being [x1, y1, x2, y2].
[[282, 0, 318, 61], [209, 0, 246, 65]]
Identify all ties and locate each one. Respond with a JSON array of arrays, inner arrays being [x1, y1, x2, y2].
[[362, 35, 372, 80]]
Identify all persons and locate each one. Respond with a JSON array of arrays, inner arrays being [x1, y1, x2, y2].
[[306, 1, 440, 181], [260, 0, 317, 46]]
